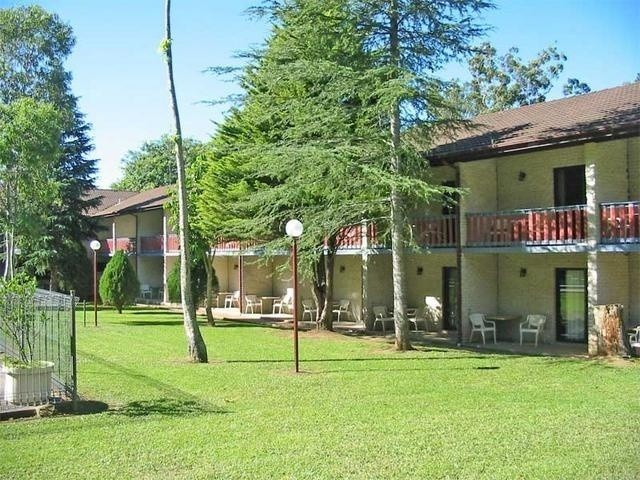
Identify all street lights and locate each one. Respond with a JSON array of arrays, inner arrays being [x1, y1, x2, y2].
[[284, 219, 305, 372], [90, 239, 101, 327], [14, 248, 22, 276]]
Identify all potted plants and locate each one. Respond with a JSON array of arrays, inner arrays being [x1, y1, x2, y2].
[[0, 271, 58, 407]]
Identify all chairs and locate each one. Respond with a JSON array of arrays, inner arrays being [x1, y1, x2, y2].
[[272, 294, 291, 314], [333, 298, 351, 322], [625, 320, 640, 346], [70, 289, 80, 304], [301, 298, 317, 322], [519, 314, 547, 347], [140, 283, 153, 299], [406, 303, 431, 333], [468, 313, 498, 346], [371, 304, 395, 333], [158, 286, 165, 300], [244, 293, 263, 315], [483, 210, 639, 243], [224, 290, 241, 309], [203, 287, 221, 308]]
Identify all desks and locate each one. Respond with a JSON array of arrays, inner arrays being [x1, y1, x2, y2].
[[217, 291, 234, 295], [261, 296, 281, 300], [389, 306, 417, 312], [485, 315, 521, 322]]
